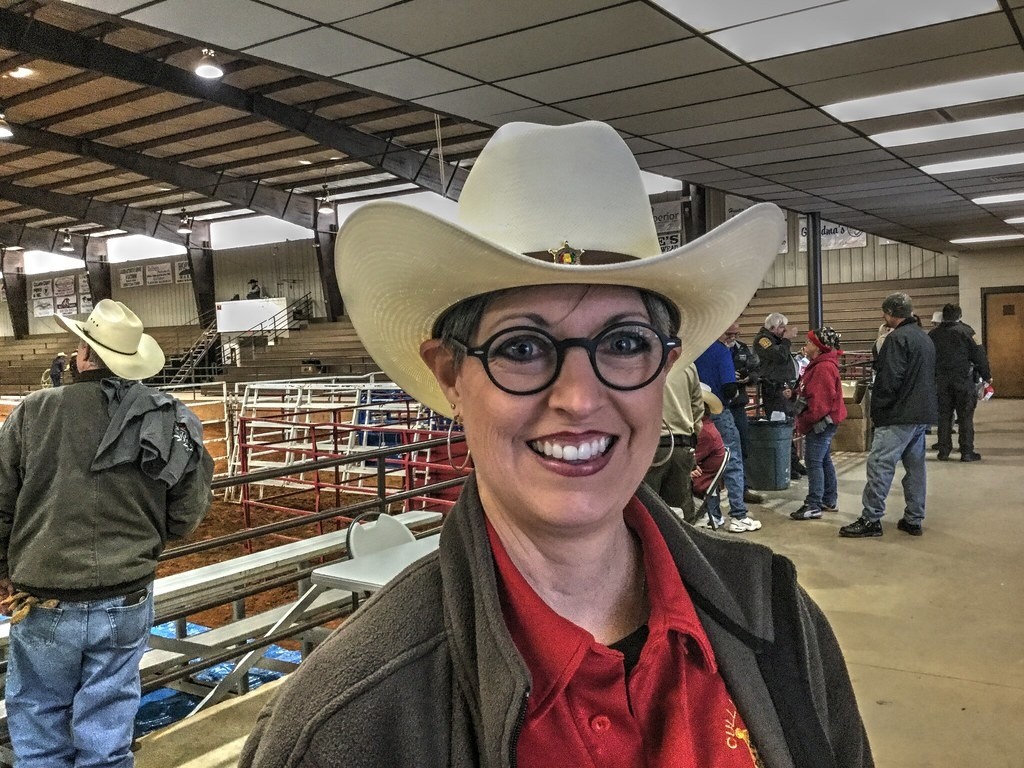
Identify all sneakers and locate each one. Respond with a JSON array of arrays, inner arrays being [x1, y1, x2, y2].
[[729, 517, 761, 533], [743, 488, 765, 503], [789, 504, 822, 519], [897, 519, 921, 535], [839, 519, 883, 537], [706, 516, 724, 528], [818, 504, 838, 512], [961, 452, 980, 461], [937, 453, 948, 461]]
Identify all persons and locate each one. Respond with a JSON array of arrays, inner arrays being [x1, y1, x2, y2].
[[239, 121, 878, 768], [645, 292, 993, 537], [0, 277, 261, 768]]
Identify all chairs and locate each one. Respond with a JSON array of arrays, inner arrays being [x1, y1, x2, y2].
[[346, 511, 417, 600], [690, 447, 731, 531]]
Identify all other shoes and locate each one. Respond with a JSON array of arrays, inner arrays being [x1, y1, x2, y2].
[[791, 466, 802, 480], [932, 442, 938, 449], [951, 429, 956, 434], [955, 420, 958, 423], [925, 430, 931, 434], [794, 461, 806, 475]]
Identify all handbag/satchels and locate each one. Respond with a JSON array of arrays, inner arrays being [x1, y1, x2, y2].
[[786, 395, 807, 416], [982, 380, 994, 402]]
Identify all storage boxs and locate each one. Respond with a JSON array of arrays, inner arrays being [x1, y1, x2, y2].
[[830, 380, 874, 451]]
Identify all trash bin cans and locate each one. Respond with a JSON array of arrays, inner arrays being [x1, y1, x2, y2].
[[745, 415, 795, 491], [303, 360, 320, 372]]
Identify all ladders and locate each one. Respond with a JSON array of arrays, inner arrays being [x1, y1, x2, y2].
[[163, 322, 219, 393]]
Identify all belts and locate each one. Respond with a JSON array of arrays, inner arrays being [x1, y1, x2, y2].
[[660, 435, 691, 447]]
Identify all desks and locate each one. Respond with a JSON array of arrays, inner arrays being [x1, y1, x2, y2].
[[311, 529, 442, 593]]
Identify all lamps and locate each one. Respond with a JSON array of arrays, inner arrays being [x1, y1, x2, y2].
[[194, 47, 223, 79], [318, 165, 335, 214], [177, 192, 192, 234], [60, 215, 74, 252], [0, 113, 14, 138]]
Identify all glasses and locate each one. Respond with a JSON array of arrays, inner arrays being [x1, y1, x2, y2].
[[448, 325, 681, 397], [724, 331, 740, 338]]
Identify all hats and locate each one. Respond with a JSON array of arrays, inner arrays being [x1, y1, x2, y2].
[[697, 381, 723, 415], [808, 326, 843, 357], [56, 352, 68, 357], [931, 311, 943, 322], [53, 299, 166, 380], [68, 353, 79, 359], [334, 113, 788, 419]]
